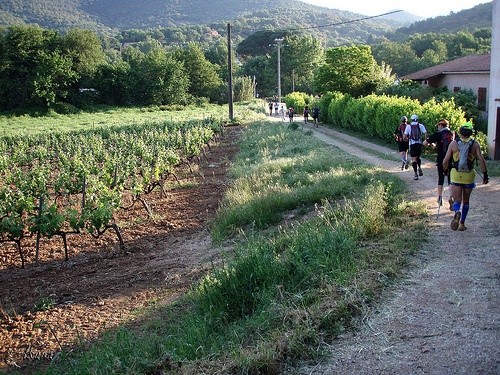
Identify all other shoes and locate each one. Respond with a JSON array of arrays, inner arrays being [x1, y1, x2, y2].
[[414, 176, 417, 179], [405, 161, 409, 169], [418, 168, 423, 176], [402, 162, 405, 170], [458, 223, 466, 231], [449, 198, 455, 211], [437, 196, 442, 206], [452, 210, 461, 231]]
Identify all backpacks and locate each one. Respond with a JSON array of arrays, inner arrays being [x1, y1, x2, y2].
[[456, 138, 477, 172], [439, 131, 454, 153], [409, 123, 421, 140]]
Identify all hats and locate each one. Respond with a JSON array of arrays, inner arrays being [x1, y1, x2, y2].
[[434, 121, 449, 126], [411, 115, 418, 120]]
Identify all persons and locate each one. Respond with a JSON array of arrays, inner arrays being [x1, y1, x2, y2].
[[304, 106, 310, 124], [392, 116, 409, 170], [443, 122, 488, 231], [311, 106, 320, 128], [402, 115, 429, 180], [421, 118, 462, 210], [268, 101, 294, 123]]
